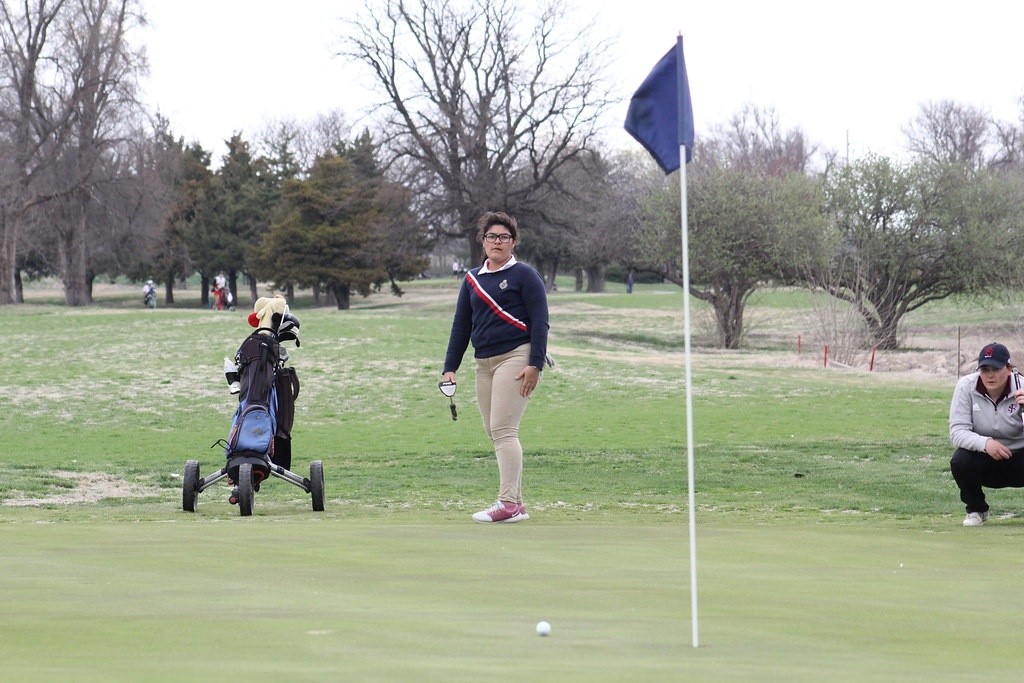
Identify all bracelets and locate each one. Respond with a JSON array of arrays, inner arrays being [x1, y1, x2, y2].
[[531, 365, 541, 371]]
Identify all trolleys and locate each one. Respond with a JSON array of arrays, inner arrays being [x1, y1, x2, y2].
[[180, 315, 327, 517]]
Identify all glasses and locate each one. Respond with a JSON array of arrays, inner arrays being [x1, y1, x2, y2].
[[484, 233, 512, 244]]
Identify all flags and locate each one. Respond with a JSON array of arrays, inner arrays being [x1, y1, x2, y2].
[[624, 35, 695, 175]]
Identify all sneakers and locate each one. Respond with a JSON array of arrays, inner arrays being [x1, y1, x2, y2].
[[963, 511, 988, 527], [518, 505, 530, 520], [471, 501, 522, 523]]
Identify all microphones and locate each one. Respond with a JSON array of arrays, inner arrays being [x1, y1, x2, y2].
[[1013, 368, 1023, 407]]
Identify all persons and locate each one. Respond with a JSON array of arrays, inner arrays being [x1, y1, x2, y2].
[[417, 271, 431, 279], [949, 340, 1024, 528], [211, 270, 229, 311], [212, 283, 224, 310], [457, 259, 465, 280], [451, 259, 460, 279], [142, 279, 156, 307], [441, 211, 551, 526], [625, 273, 634, 293]]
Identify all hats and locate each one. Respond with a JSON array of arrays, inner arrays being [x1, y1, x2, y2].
[[975, 342, 1010, 371]]
